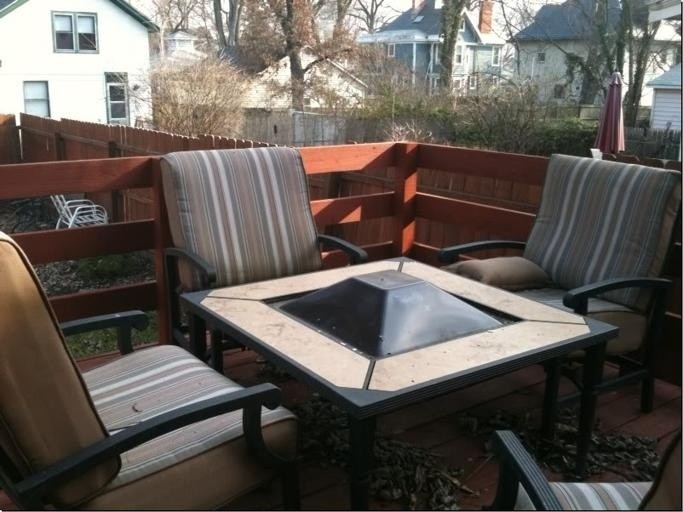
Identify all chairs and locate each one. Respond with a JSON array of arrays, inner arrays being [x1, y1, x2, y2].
[[492, 428, 683, 508], [438, 154, 681, 476], [159, 149, 370, 371], [1, 230, 303, 509]]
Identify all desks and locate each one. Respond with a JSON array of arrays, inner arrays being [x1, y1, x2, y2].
[[178, 256, 619, 512]]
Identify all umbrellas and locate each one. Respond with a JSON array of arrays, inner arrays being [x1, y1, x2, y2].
[[592, 72, 627, 156]]
